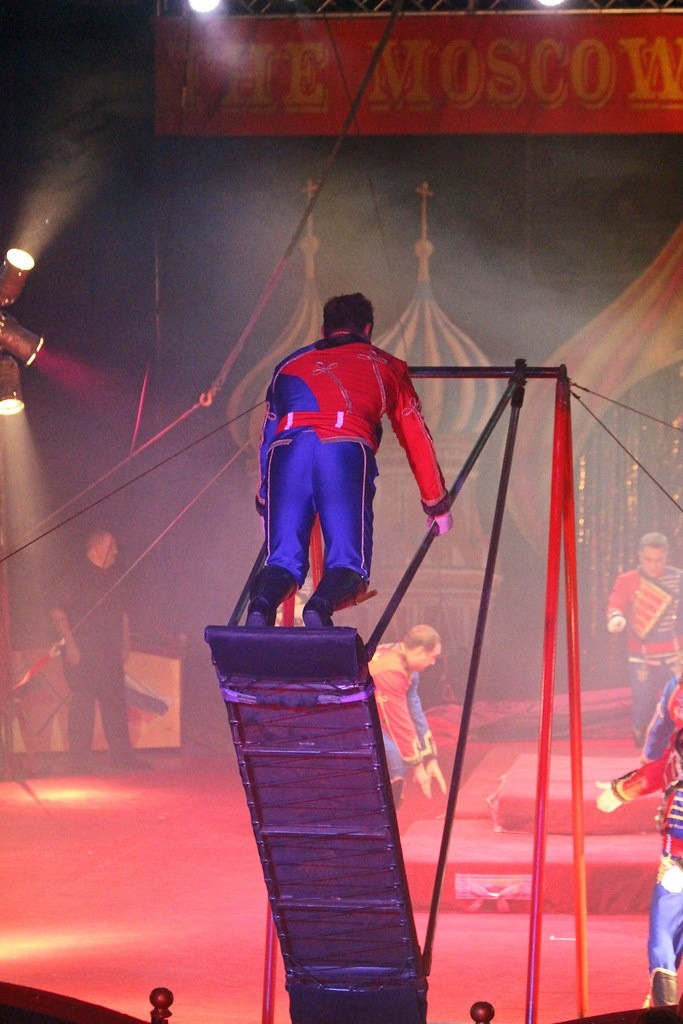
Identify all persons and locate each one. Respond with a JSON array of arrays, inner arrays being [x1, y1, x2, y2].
[[245, 292, 454, 627], [51, 526, 150, 771], [367, 625, 448, 809], [595, 532, 683, 1008]]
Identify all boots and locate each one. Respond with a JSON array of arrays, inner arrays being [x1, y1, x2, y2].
[[245, 563, 300, 628], [649, 967, 678, 1007], [303, 565, 368, 628]]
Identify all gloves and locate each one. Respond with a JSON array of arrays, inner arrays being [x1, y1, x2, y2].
[[413, 759, 448, 798], [594, 779, 623, 814], [427, 509, 453, 535], [606, 615, 626, 633]]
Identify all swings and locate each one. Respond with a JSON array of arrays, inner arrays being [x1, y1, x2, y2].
[[201, 360, 526, 1024]]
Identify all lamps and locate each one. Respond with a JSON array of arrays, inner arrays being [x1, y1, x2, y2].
[[0, 311, 44, 367], [0, 355, 26, 415], [0, 247, 35, 308]]
